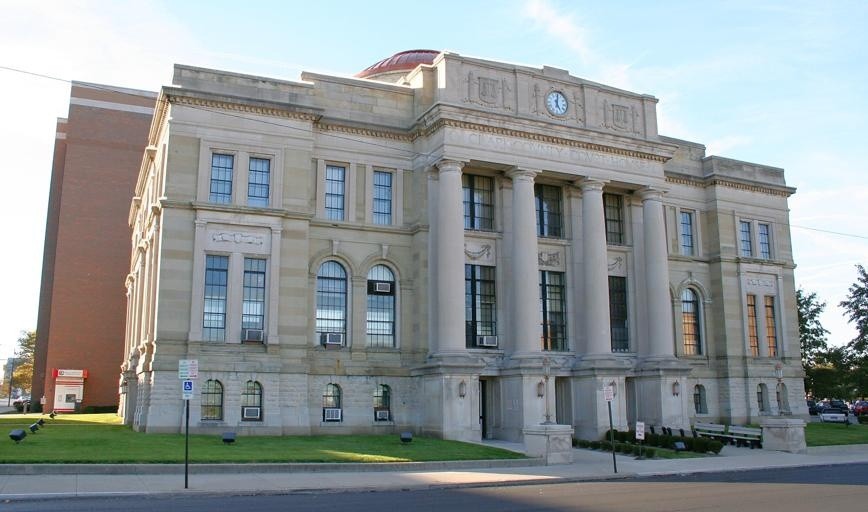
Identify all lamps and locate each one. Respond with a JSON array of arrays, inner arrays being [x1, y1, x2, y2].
[[458, 379, 468, 399], [773, 361, 787, 383], [609, 380, 617, 397], [673, 380, 680, 397], [537, 380, 545, 397]]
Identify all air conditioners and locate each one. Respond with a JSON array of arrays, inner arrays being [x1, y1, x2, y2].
[[323, 408, 342, 423], [373, 283, 391, 292], [245, 329, 263, 340], [243, 408, 260, 419], [321, 332, 343, 345], [476, 335, 498, 347], [375, 410, 389, 421]]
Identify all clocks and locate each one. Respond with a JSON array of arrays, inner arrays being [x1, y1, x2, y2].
[[545, 90, 567, 116]]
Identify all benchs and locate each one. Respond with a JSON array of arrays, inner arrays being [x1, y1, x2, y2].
[[651, 419, 761, 456]]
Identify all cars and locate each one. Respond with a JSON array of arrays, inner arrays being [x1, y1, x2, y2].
[[807, 398, 868, 423], [13, 396, 31, 406]]
